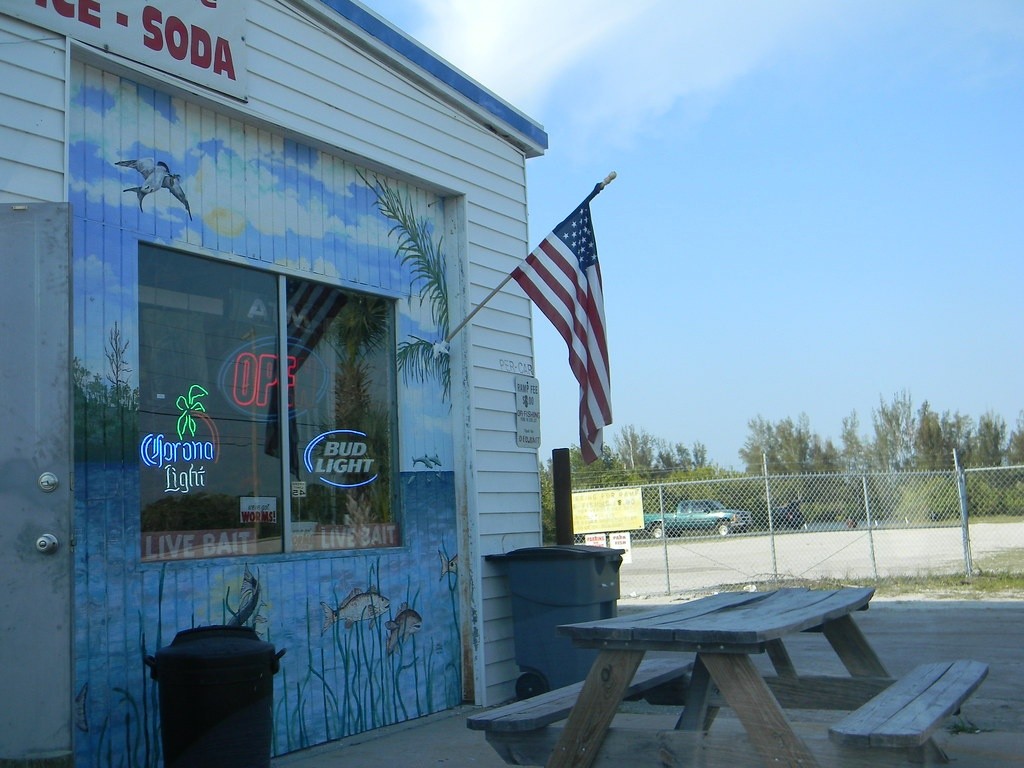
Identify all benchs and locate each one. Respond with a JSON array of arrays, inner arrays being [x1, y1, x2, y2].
[[827, 662, 990, 767], [466, 658, 694, 768]]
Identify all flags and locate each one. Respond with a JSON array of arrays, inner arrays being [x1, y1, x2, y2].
[[510, 199, 612, 464]]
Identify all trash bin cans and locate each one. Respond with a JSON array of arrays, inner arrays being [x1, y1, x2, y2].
[[482, 543, 627, 704], [144, 622, 291, 768]]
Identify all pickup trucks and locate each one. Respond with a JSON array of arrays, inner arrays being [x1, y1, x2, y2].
[[644, 499, 752, 537]]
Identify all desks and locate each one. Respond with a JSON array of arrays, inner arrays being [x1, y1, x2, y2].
[[547, 587, 895, 768]]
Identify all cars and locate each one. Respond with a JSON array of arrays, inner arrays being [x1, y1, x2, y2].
[[767, 498, 858, 528]]
